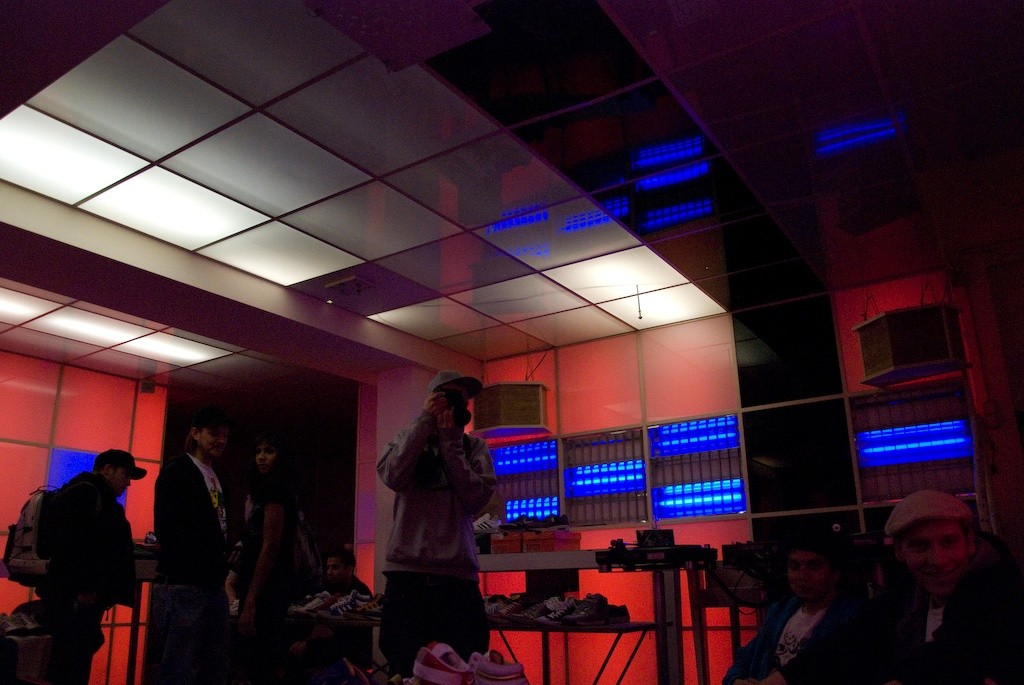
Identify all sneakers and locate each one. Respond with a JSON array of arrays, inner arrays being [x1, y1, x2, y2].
[[485, 594, 521, 615], [535, 598, 583, 626], [560, 593, 607, 625], [404, 640, 476, 685], [342, 593, 387, 621], [0, 614, 39, 640], [487, 600, 534, 624], [526, 513, 571, 532], [471, 514, 504, 534], [316, 591, 371, 619], [498, 516, 539, 532], [16, 611, 52, 639], [606, 603, 629, 624], [510, 597, 565, 625], [468, 651, 528, 685], [285, 591, 334, 616]]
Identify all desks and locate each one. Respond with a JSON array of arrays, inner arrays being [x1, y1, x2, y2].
[[686, 559, 765, 685], [475, 542, 719, 685]]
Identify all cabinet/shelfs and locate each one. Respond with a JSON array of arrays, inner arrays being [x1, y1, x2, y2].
[[484, 372, 995, 546]]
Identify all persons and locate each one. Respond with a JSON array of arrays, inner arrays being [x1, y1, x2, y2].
[[221, 429, 374, 685], [35, 449, 147, 685], [722, 525, 867, 685], [750, 489, 1023, 685], [143, 405, 232, 685], [374, 369, 502, 684]]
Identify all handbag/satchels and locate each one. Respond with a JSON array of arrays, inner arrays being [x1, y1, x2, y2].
[[293, 519, 323, 580]]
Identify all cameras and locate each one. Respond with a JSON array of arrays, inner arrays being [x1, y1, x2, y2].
[[443, 390, 472, 427]]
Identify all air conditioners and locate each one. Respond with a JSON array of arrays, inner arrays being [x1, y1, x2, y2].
[[849, 302, 970, 387], [469, 380, 555, 439]]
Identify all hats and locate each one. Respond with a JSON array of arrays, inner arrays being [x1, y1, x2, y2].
[[883, 492, 973, 536], [428, 370, 483, 398], [95, 448, 147, 479], [193, 407, 237, 428]]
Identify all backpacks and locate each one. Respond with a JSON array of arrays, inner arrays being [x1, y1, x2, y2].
[[3, 481, 100, 585]]
